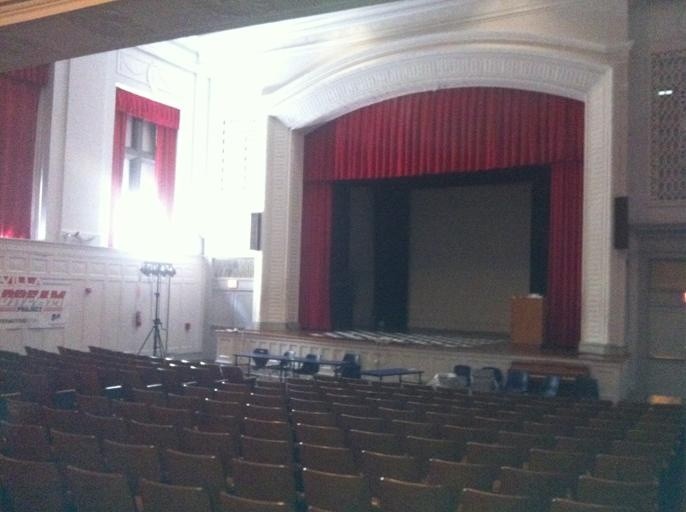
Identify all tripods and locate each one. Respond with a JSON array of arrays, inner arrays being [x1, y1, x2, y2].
[[137, 274, 168, 357]]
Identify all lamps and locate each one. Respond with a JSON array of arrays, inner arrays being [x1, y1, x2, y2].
[[136, 260, 176, 358]]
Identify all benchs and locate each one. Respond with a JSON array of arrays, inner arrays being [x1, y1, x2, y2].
[[0, 346, 686, 512]]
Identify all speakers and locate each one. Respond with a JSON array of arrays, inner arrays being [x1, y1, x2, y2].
[[615, 197, 628, 249], [250, 213, 262, 251]]
